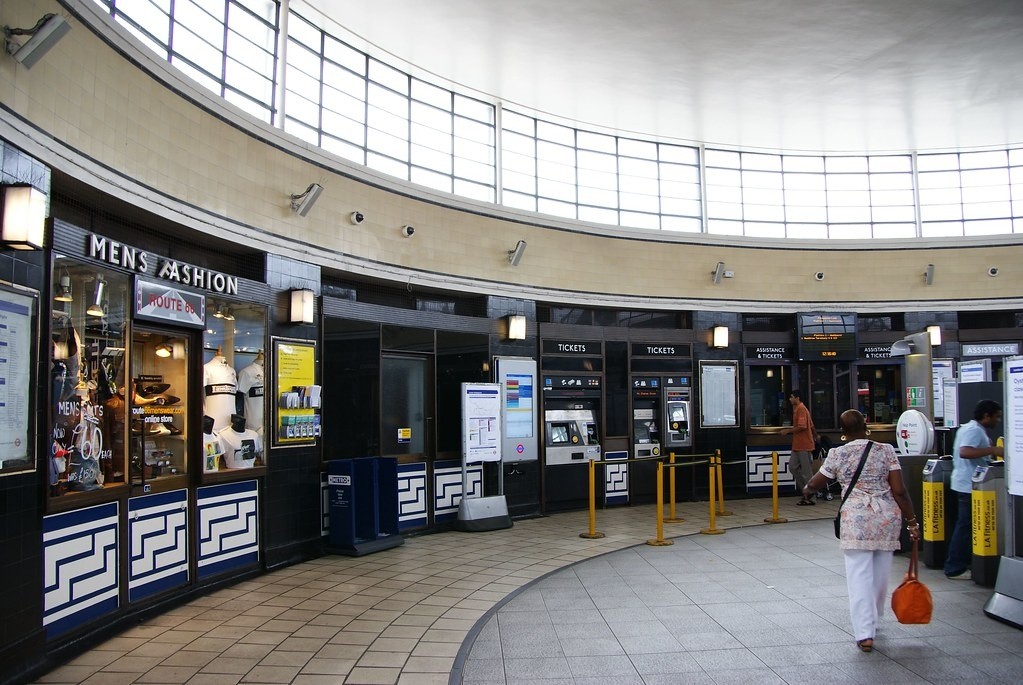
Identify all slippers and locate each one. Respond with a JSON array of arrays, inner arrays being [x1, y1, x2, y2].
[[796, 492, 815, 505]]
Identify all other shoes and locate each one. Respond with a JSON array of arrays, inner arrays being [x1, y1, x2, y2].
[[949, 569, 972, 579]]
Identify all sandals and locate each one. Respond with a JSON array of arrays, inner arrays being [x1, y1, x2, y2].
[[858, 638, 873, 652]]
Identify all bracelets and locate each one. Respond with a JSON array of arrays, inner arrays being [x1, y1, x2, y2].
[[906, 523, 919, 530], [904, 515, 916, 522]]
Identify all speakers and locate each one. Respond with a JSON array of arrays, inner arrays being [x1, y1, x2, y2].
[[926, 264, 935, 284], [510, 240, 526, 266], [713, 262, 724, 284], [296, 183, 324, 217], [11, 13, 71, 69]]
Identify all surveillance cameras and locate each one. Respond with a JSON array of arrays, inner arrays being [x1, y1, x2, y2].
[[988, 267, 998, 277], [351, 212, 364, 225], [401, 225, 415, 238], [815, 272, 824, 280]]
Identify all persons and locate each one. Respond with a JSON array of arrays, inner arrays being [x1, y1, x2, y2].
[[202, 355, 264, 472], [780, 390, 820, 505], [944, 399, 1004, 579], [803, 409, 920, 652]]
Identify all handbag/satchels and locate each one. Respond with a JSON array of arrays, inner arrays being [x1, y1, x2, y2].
[[891, 540, 932, 624], [833, 516, 841, 540]]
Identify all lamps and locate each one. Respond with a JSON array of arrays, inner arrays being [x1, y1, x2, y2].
[[712, 325, 728, 348], [927, 324, 942, 345], [55, 263, 73, 301], [87, 273, 107, 318], [288, 286, 314, 325], [226, 302, 236, 321], [212, 300, 224, 319], [0, 180, 49, 251], [508, 314, 527, 339], [154, 343, 172, 357]]
